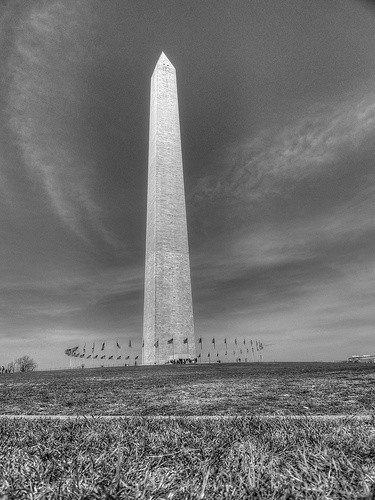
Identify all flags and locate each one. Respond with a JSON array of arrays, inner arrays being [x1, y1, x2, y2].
[[63, 337, 265, 364]]
